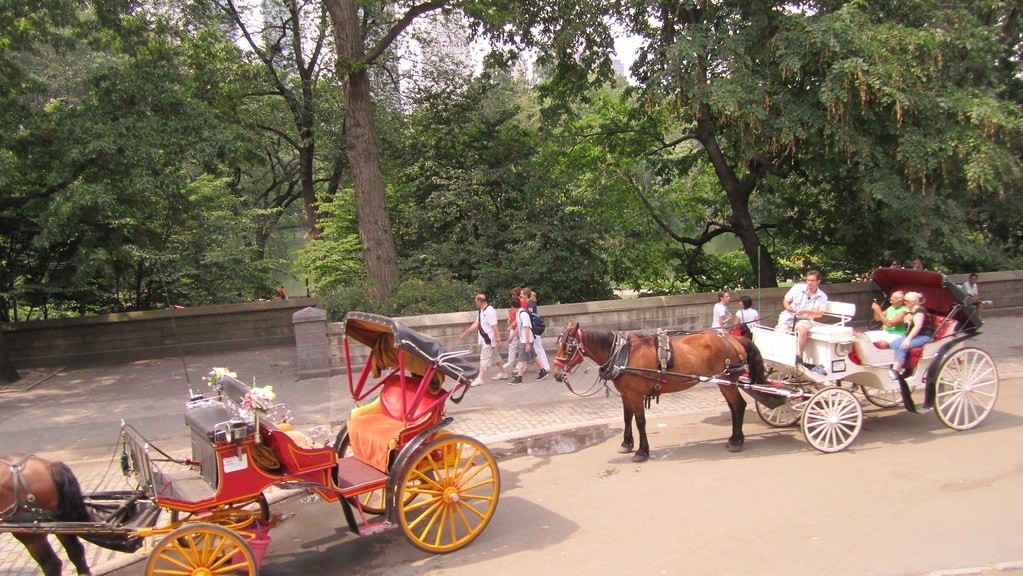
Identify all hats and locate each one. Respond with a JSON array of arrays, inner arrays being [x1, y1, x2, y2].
[[903, 259, 913, 268]]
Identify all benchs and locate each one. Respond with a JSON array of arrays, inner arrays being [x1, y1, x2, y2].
[[182, 371, 338, 491], [852, 313, 958, 366], [346, 374, 447, 475], [795, 300, 857, 343]]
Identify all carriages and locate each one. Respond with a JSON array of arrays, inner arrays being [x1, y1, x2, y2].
[[552, 268, 1000, 454], [1, 311, 501, 576]]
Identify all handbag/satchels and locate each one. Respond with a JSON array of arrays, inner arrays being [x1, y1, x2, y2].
[[734, 311, 751, 340]]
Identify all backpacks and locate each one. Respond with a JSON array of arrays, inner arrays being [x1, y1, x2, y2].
[[519, 310, 545, 335]]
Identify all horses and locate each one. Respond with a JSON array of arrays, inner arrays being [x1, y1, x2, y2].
[[552, 320, 766, 463], [0, 454, 92, 576]]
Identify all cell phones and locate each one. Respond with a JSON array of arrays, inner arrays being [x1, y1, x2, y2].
[[873, 299, 877, 303]]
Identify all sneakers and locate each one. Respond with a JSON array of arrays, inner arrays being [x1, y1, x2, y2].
[[535, 369, 548, 382], [470, 378, 484, 387], [508, 377, 522, 385], [492, 371, 508, 379]]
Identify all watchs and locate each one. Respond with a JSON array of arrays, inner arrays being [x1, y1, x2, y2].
[[526, 341, 530, 344], [497, 339, 500, 342]]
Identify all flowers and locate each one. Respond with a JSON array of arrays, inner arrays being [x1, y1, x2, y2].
[[201, 367, 238, 393], [239, 385, 277, 412]]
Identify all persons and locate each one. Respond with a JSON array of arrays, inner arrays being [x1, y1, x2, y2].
[[460, 294, 508, 386], [699, 291, 732, 382], [890, 259, 922, 271], [734, 296, 760, 340], [889, 292, 933, 379], [503, 287, 550, 385], [961, 273, 992, 323], [774, 269, 828, 366], [864, 291, 911, 350]]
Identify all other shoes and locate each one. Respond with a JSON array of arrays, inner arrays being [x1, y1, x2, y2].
[[537, 369, 551, 374], [513, 367, 527, 374], [982, 300, 992, 305], [981, 320, 985, 323], [503, 363, 514, 369], [888, 368, 906, 378]]
